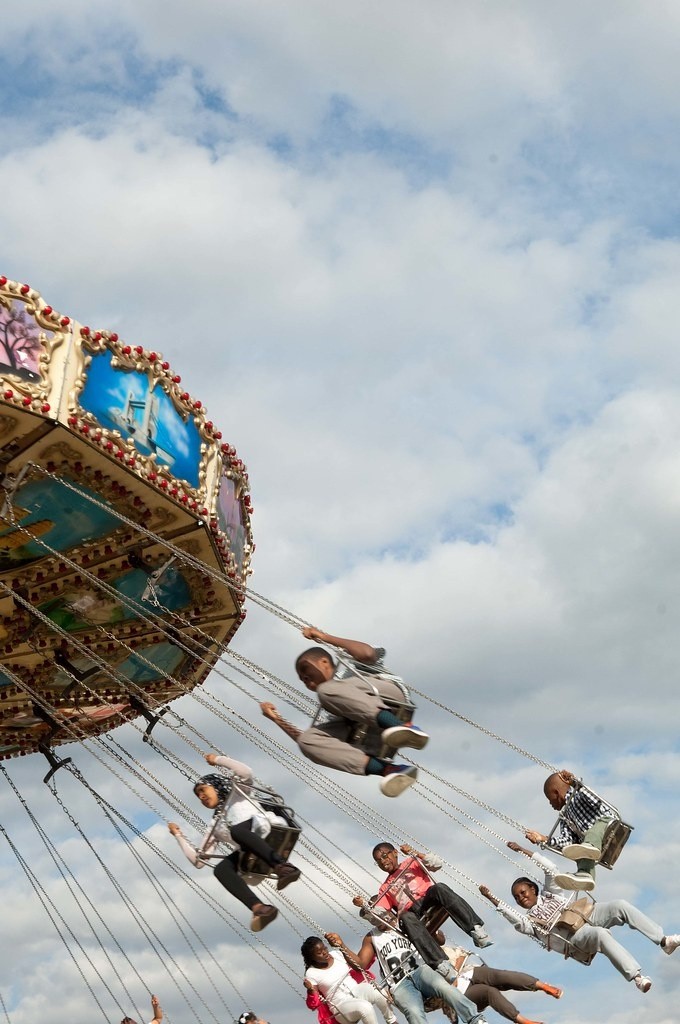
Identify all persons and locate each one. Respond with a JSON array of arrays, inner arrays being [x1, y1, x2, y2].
[[120, 995, 163, 1024], [479, 842, 680, 993], [168, 753, 301, 932], [239, 1012, 270, 1024], [352, 842, 493, 984], [259, 626, 430, 797], [525, 770, 617, 891], [301, 894, 564, 1024]]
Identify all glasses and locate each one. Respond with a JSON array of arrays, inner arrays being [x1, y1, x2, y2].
[[373, 851, 392, 865]]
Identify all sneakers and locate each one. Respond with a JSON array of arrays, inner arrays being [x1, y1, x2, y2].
[[635, 975, 652, 993], [276, 863, 301, 891], [379, 764, 418, 798], [554, 871, 595, 891], [382, 722, 428, 750], [435, 960, 458, 985], [562, 843, 601, 860], [471, 925, 494, 949], [249, 905, 278, 932], [661, 934, 680, 955]]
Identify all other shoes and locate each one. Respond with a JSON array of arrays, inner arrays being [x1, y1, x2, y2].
[[524, 1020, 546, 1024], [544, 984, 564, 999]]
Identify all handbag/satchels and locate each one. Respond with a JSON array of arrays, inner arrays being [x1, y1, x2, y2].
[[557, 897, 595, 933]]
